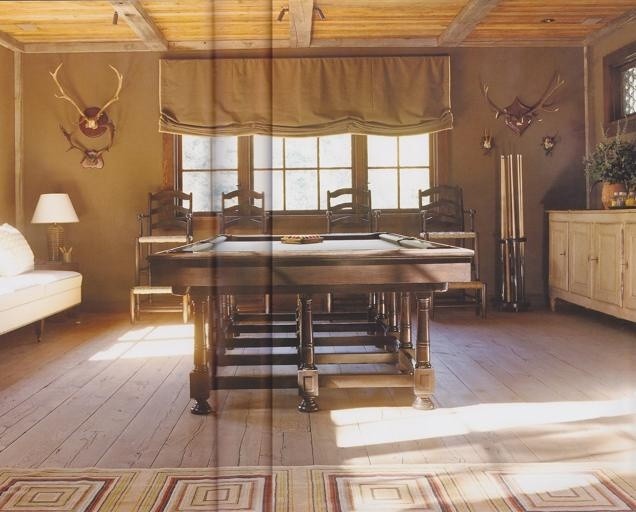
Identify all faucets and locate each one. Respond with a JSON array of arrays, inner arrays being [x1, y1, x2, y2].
[[589, 179, 602, 193]]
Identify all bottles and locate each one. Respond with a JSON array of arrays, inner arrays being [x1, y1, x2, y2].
[[611, 191, 636, 208]]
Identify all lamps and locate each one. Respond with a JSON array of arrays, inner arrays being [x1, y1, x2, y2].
[[29, 193, 80, 264]]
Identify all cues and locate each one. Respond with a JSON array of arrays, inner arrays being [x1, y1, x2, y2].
[[499, 153, 526, 301]]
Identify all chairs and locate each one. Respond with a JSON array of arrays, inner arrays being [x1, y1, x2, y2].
[[418, 183, 488, 322], [0, 222, 83, 343], [127, 189, 193, 324], [325, 185, 381, 233], [216, 189, 273, 237]]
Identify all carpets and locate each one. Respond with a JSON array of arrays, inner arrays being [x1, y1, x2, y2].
[[1, 465, 635, 511]]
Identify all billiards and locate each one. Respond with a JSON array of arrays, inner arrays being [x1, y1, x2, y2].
[[283, 235, 319, 239]]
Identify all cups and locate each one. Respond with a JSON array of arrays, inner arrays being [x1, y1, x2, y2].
[[62, 252, 71, 263]]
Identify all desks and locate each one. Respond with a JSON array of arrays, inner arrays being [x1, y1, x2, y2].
[[145, 233, 475, 413]]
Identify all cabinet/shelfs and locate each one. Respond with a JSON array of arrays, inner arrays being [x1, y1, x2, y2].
[[543, 206, 636, 324]]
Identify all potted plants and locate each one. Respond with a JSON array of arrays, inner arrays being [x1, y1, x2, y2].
[[579, 111, 636, 209]]
